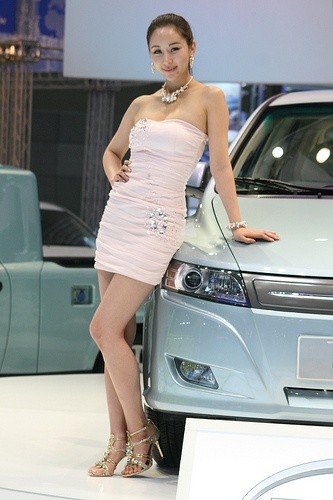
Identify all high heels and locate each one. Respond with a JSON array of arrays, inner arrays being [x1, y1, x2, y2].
[[88, 434, 127, 477], [122, 419, 164, 477]]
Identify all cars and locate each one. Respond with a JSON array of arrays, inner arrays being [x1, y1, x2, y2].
[[141, 89, 333, 467]]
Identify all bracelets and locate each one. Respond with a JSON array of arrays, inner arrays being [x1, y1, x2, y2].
[[226, 222, 247, 229]]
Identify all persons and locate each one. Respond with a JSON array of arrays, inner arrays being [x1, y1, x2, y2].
[[88, 13, 279, 477]]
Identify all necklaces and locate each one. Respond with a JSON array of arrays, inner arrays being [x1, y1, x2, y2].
[[162, 76, 194, 103]]
